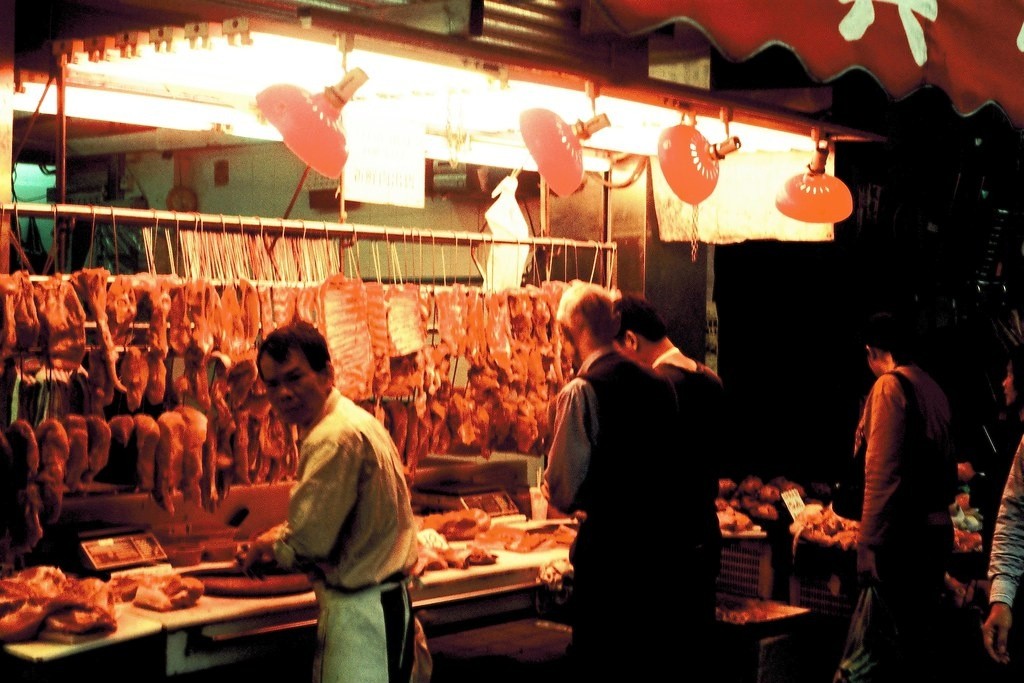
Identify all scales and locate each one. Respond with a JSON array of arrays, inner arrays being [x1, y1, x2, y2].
[[24, 520, 168, 572]]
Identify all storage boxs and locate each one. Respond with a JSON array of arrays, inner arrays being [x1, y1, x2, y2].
[[718, 543, 857, 623]]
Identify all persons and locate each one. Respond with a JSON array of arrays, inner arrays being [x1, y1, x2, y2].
[[540, 282, 723, 683], [233, 322, 433, 683], [834, 314, 1024, 683]]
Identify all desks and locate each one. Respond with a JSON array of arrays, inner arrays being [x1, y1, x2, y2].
[[4, 538, 571, 682]]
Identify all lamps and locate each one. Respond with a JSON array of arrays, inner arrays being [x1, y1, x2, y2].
[[519, 79, 610, 200], [660, 107, 742, 208], [774, 127, 852, 223], [256, 30, 368, 180]]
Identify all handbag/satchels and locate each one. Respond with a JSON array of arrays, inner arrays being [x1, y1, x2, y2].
[[832, 370, 941, 525], [834, 585, 903, 683]]
